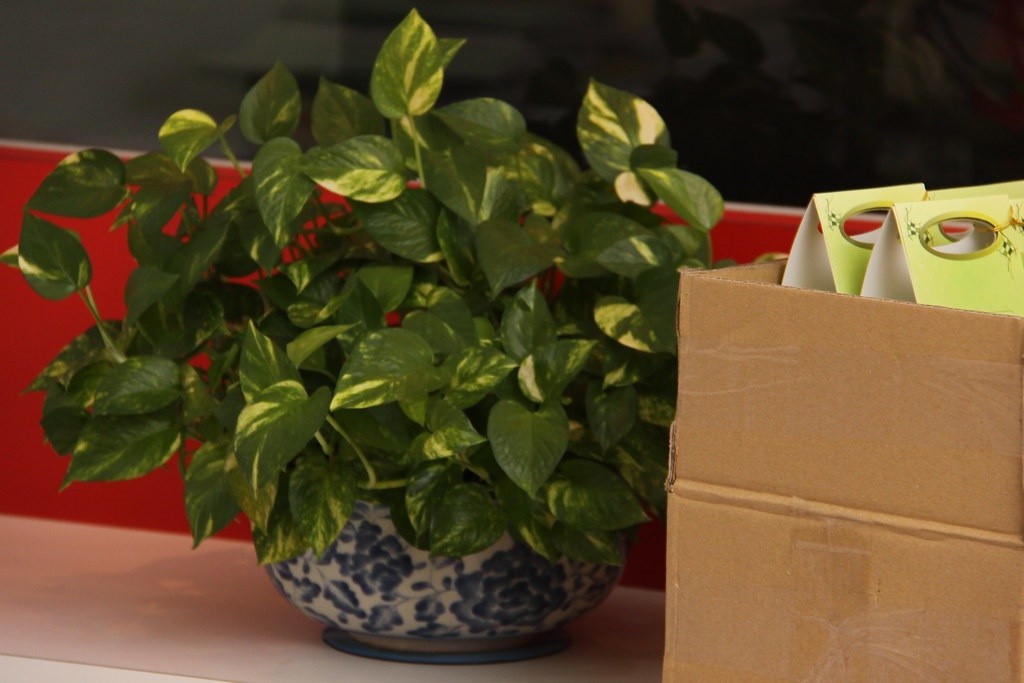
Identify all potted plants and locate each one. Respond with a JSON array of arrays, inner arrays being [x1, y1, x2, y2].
[[1, 6, 790, 665]]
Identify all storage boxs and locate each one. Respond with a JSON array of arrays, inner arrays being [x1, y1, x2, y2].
[[663, 251, 1024, 682]]
